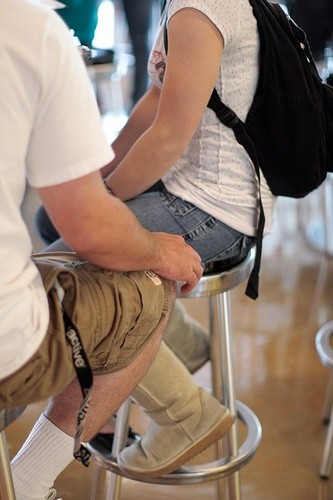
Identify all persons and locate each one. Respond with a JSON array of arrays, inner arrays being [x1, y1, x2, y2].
[[0, 0, 203, 500], [35, 0, 277, 481]]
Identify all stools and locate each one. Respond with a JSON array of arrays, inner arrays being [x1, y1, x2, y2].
[[0, 173, 332, 500]]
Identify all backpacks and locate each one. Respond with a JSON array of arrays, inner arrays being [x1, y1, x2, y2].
[[164, 0, 333, 199]]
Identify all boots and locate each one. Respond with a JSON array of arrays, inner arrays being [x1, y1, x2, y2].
[[117, 342, 235, 480], [161, 300, 210, 374]]
